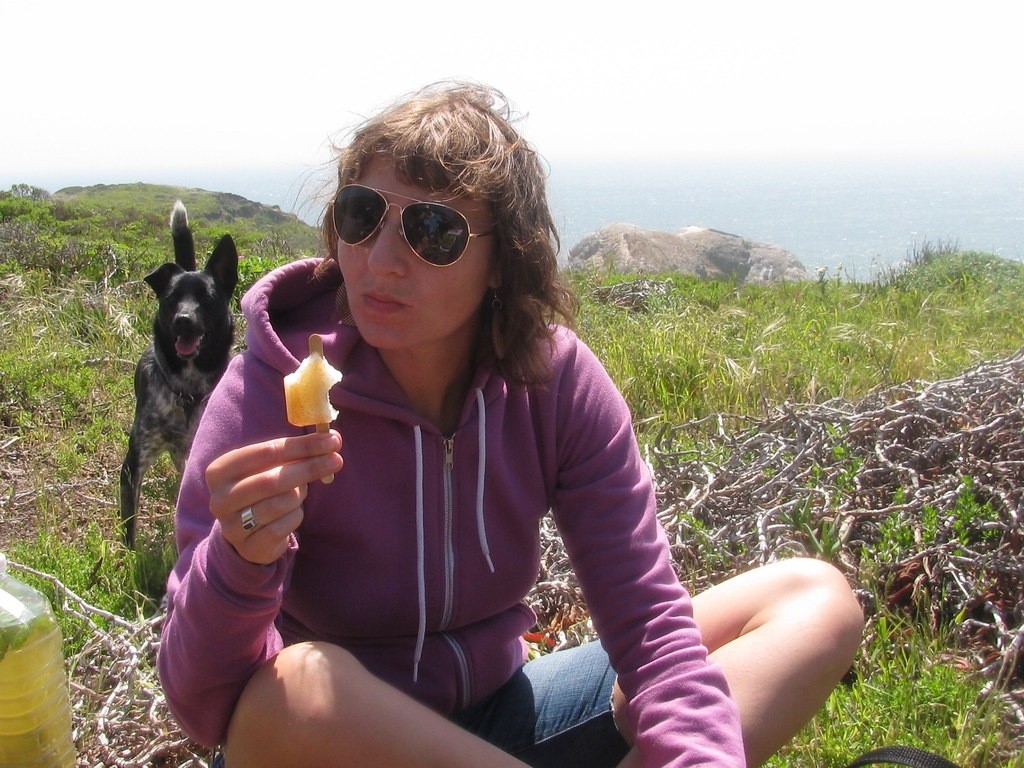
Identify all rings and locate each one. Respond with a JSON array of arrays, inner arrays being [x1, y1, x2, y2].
[[241, 507, 262, 532]]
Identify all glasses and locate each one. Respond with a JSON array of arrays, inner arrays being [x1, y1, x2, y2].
[[329, 184, 496, 268]]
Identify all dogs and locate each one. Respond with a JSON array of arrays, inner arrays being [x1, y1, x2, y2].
[[119, 198, 238, 552]]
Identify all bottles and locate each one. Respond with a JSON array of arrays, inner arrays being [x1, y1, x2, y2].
[[0, 552, 77, 768]]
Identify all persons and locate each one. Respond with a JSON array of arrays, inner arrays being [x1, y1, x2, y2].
[[156, 82, 866, 767]]
